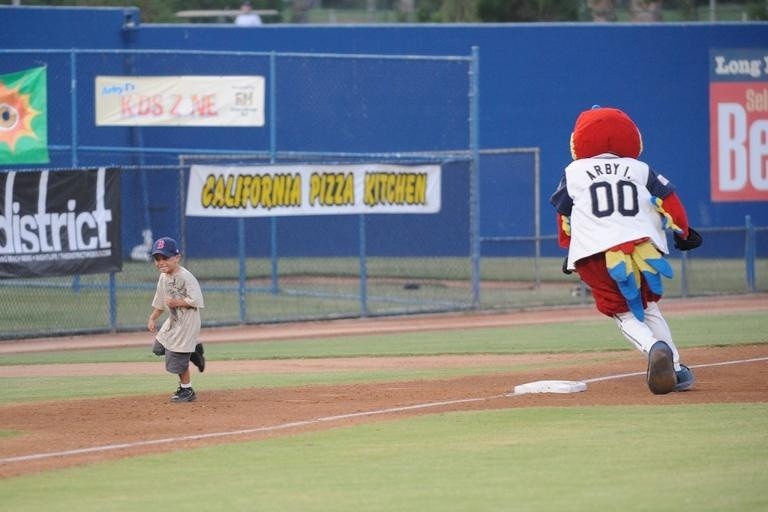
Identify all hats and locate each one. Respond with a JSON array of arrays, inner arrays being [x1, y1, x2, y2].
[[151, 237, 180, 260]]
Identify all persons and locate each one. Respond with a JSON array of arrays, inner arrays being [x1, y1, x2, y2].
[[147, 237, 206, 402], [233, 0, 265, 27], [547, 104, 703, 395]]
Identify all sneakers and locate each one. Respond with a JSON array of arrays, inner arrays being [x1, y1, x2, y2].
[[189, 343, 206, 372], [170, 385, 197, 402]]
